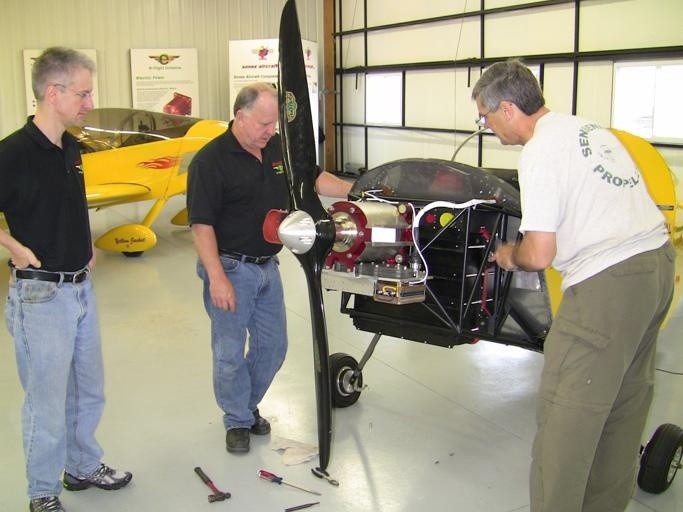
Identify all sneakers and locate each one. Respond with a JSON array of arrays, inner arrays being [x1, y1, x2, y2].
[[30, 495, 66, 512], [64, 463, 133, 491], [250, 409, 271, 435], [226, 428, 250, 453]]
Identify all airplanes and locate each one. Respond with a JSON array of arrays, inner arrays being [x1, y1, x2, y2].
[[0, 108, 289, 257], [278, 1, 683, 494]]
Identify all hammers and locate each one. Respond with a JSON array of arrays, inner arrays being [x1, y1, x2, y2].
[[194, 467, 231, 503]]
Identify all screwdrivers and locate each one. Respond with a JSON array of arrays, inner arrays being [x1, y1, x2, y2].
[[255, 469, 322, 496], [284, 502, 320, 512]]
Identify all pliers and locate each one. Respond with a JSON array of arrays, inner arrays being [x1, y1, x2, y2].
[[311, 467, 339, 487]]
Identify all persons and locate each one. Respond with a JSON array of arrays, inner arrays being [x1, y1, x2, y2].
[[470, 56, 677, 512], [0, 45, 134, 512], [185, 81, 353, 453]]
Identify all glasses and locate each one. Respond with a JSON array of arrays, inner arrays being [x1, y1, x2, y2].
[[54, 84, 94, 101], [474, 109, 495, 128]]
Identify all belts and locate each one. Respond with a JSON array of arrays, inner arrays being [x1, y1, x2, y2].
[[10, 269, 88, 283], [219, 247, 274, 265]]
